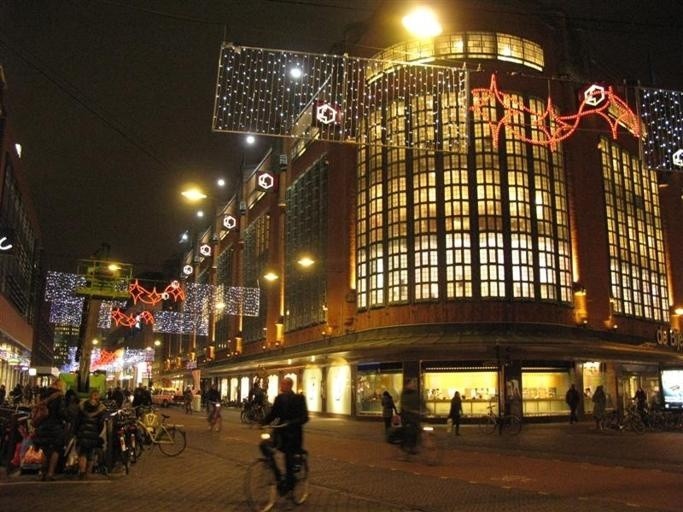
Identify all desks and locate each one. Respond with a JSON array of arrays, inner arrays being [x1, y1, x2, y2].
[[427, 397, 567, 413]]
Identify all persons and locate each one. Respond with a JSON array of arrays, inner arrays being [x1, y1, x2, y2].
[[632, 388, 647, 406], [448, 391, 463, 435], [250, 383, 263, 414], [207, 384, 222, 423], [400, 379, 433, 454], [382, 392, 397, 435], [183, 387, 193, 414], [251, 378, 308, 499], [0, 379, 153, 480], [592, 386, 607, 429], [566, 384, 579, 423]]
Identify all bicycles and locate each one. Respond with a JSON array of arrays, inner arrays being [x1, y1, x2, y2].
[[198, 400, 226, 432], [237, 397, 281, 427], [594, 400, 683, 434], [474, 403, 522, 436], [241, 419, 313, 511], [109, 399, 188, 477]]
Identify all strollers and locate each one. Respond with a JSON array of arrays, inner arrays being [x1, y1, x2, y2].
[[384, 408, 419, 448]]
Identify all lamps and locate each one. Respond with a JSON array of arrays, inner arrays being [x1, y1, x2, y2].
[[570, 281, 587, 298]]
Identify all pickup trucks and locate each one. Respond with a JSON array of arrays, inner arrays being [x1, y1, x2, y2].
[[149, 384, 182, 407]]
